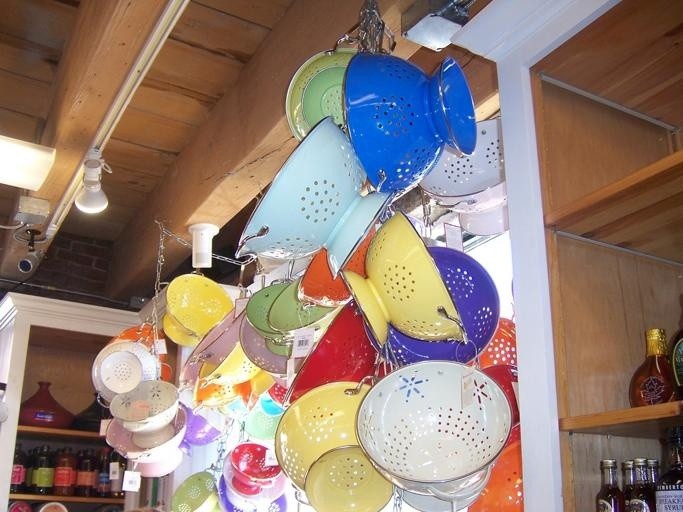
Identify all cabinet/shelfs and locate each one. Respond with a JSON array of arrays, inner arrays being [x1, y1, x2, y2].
[[448, 0, 681, 512], [0, 291, 142, 512]]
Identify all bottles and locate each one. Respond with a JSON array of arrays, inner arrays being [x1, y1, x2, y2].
[[9, 379, 126, 498], [627, 325, 683, 407], [595, 445, 683, 512]]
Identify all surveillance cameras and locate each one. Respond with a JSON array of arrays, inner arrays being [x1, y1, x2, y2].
[[17, 249, 46, 275]]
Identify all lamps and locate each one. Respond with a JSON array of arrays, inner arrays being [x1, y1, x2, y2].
[[0, 118, 57, 193], [74, 145, 113, 215]]
